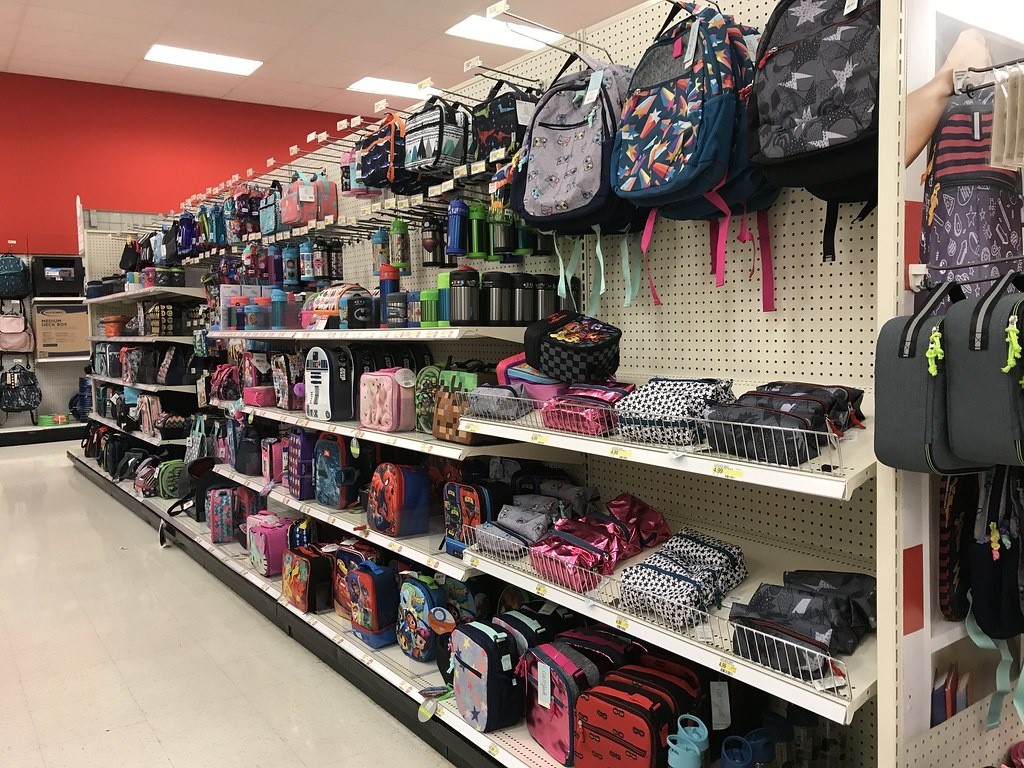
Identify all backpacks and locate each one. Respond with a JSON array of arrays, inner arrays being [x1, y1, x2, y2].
[[0, 253, 42, 414]]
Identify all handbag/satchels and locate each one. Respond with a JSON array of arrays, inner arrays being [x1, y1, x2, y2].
[[729, 568, 878, 682], [748, 0, 881, 261], [613, 378, 738, 445], [82, 0, 873, 765], [615, 527, 751, 633], [704, 381, 868, 466], [874, 266, 1024, 733]]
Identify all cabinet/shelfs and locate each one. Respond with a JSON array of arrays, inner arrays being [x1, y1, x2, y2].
[[66, 229, 211, 553], [28, 254, 88, 443], [190, 0, 1024, 768]]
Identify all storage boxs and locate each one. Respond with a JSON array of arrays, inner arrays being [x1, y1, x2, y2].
[[31, 303, 90, 354]]
